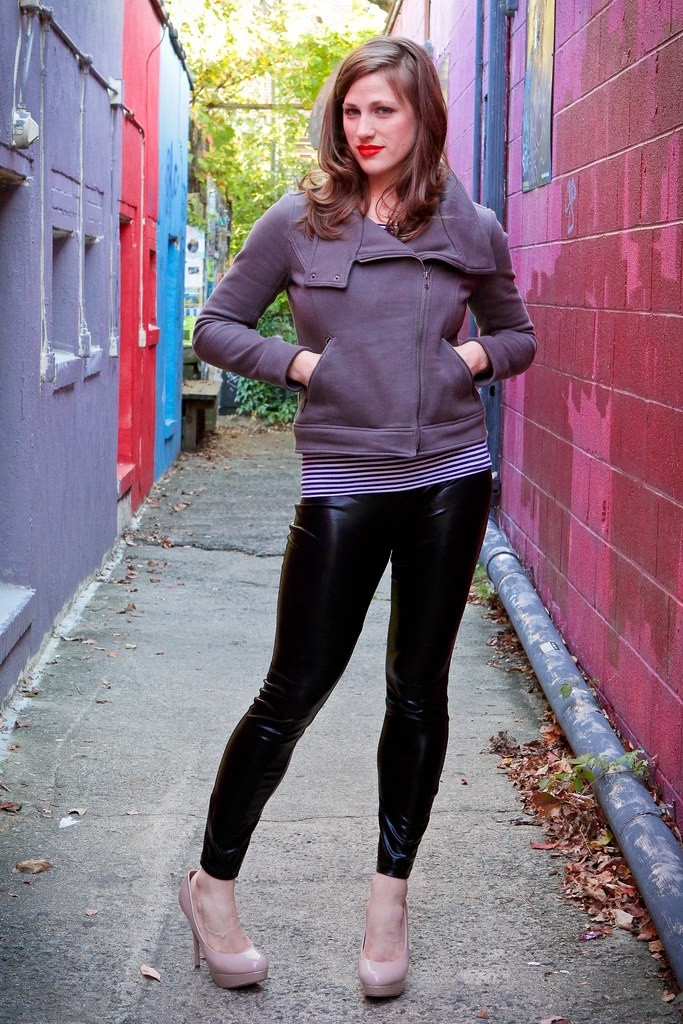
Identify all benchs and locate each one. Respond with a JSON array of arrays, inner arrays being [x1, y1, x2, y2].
[[181, 378, 223, 448]]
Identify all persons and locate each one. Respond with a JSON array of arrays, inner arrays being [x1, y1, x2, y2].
[[177, 37, 537, 996]]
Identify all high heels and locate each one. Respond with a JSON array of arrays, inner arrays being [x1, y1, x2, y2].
[[358, 902, 410, 1000], [178, 867, 268, 988]]
